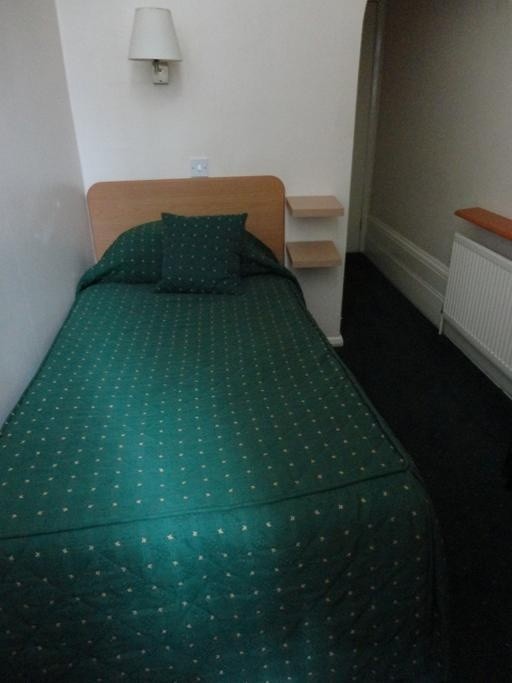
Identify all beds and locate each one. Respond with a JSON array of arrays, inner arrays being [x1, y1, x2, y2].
[[0, 175, 452, 683]]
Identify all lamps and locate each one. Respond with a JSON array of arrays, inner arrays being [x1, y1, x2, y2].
[[128, 7, 183, 85]]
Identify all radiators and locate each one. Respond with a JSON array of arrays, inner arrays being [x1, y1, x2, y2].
[[439, 230, 512, 402]]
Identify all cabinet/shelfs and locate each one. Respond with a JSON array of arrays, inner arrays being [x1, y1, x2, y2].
[[285, 195, 345, 268]]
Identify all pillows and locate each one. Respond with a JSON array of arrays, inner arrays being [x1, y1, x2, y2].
[[151, 212, 248, 298], [129, 249, 270, 284], [76, 219, 307, 310]]
